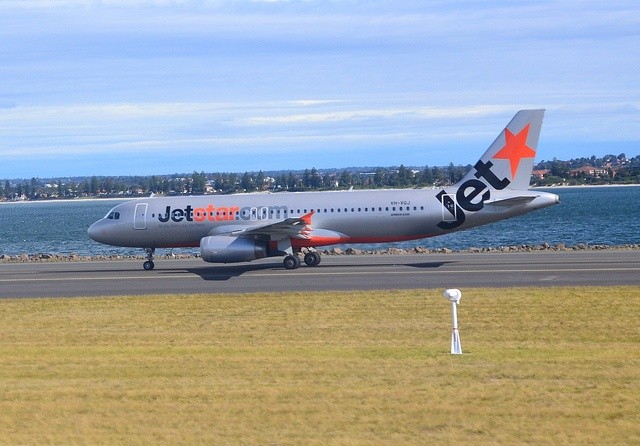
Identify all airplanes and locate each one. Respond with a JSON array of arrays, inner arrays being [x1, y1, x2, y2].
[[87, 109, 559, 270]]
